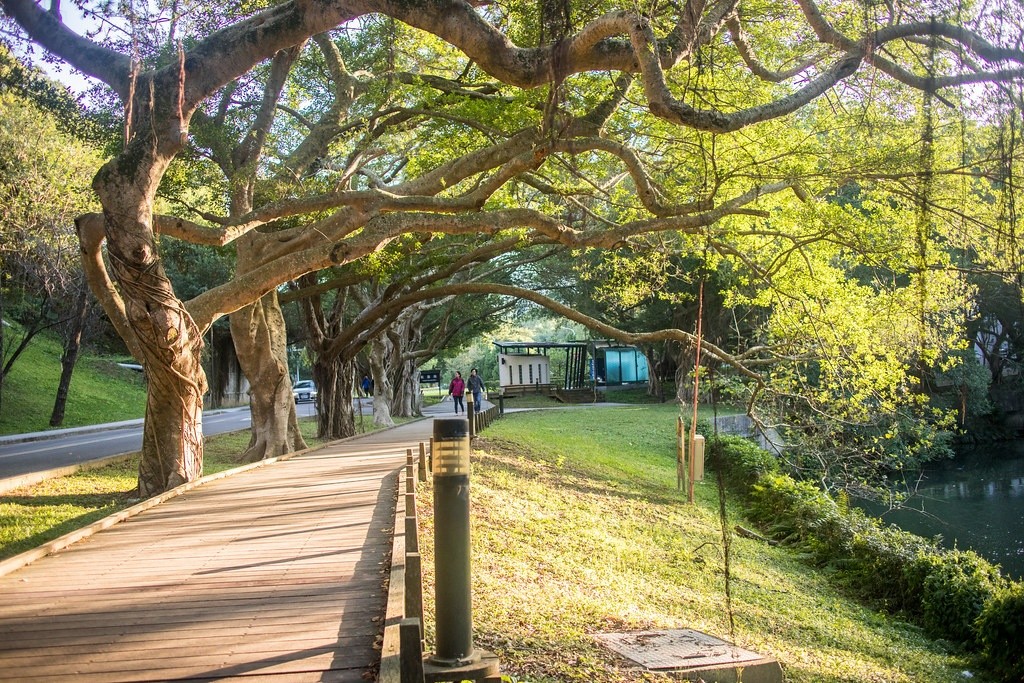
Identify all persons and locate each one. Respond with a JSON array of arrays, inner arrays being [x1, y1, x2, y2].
[[361, 376, 370, 397], [449, 371, 465, 416], [467, 369, 486, 413]]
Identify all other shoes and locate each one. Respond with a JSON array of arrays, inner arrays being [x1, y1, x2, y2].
[[462, 412, 465, 416], [455, 413, 458, 416]]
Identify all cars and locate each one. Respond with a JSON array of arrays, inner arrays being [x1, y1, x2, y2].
[[291, 380, 319, 404]]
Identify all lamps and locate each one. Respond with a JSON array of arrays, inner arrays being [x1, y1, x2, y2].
[[426, 416, 485, 668]]
